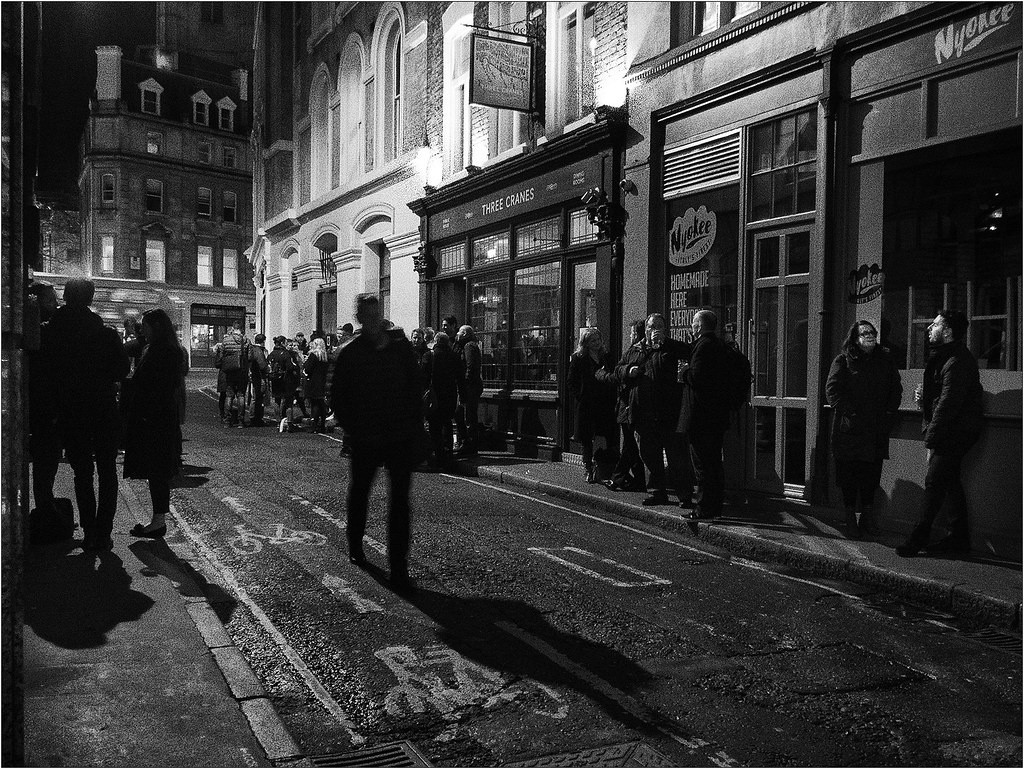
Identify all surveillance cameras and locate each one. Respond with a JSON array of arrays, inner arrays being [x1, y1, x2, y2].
[[417, 242, 423, 251], [619, 179, 633, 192], [580, 191, 592, 203]]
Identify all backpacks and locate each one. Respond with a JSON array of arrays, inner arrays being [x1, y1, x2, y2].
[[268, 358, 282, 381], [719, 338, 751, 399]]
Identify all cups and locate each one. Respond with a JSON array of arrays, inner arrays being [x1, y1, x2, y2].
[[677, 359, 688, 383]]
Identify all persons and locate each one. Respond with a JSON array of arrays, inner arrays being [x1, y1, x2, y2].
[[826, 320, 904, 540], [29, 280, 189, 556], [472, 317, 561, 391], [894, 309, 985, 557], [332, 292, 424, 590], [210, 316, 484, 473], [567, 310, 733, 521]]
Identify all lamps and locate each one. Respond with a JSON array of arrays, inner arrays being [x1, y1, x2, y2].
[[423, 105, 622, 195]]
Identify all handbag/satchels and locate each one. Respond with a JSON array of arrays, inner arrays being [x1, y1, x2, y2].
[[222, 356, 241, 372], [422, 389, 438, 418]]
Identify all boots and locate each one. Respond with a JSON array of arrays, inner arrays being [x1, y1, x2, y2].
[[845, 507, 859, 539], [858, 503, 879, 537]]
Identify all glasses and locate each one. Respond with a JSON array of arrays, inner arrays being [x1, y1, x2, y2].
[[859, 331, 877, 339]]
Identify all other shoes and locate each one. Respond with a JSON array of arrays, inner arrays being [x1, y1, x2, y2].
[[895, 542, 928, 558], [643, 496, 668, 506], [585, 472, 600, 484], [618, 484, 647, 492], [456, 443, 478, 458], [606, 480, 620, 492], [81, 531, 113, 554], [218, 410, 328, 434], [129, 523, 167, 537], [346, 530, 365, 563], [679, 506, 712, 522], [389, 577, 421, 602], [679, 499, 694, 509], [927, 540, 971, 551]]
